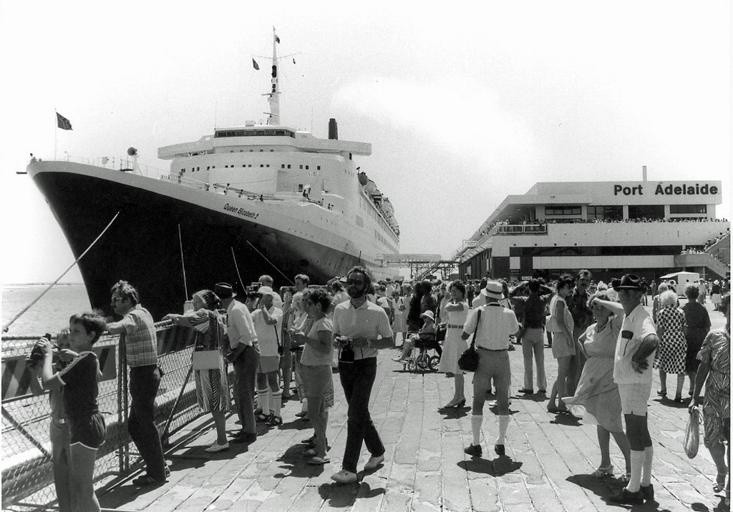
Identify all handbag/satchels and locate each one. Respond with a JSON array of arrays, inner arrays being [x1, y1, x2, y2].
[[682, 406, 703, 458], [191, 314, 223, 371], [458, 308, 481, 373]]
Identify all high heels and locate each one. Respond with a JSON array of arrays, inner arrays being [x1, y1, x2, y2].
[[657, 389, 667, 396], [462, 397, 467, 406], [444, 399, 462, 409], [559, 406, 568, 413]]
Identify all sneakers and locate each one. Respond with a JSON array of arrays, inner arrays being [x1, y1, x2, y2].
[[364, 454, 384, 471], [132, 476, 157, 483], [165, 466, 170, 476], [332, 469, 357, 483]]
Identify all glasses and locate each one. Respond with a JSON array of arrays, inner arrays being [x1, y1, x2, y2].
[[112, 296, 122, 301]]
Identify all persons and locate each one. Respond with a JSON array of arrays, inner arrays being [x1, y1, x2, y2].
[[408, 283, 424, 330], [279, 291, 294, 400], [466, 280, 476, 309], [330, 266, 394, 484], [254, 274, 282, 308], [329, 312, 340, 372], [291, 292, 316, 421], [379, 280, 383, 285], [687, 294, 730, 505], [377, 296, 392, 323], [327, 278, 336, 289], [461, 281, 519, 457], [684, 279, 690, 286], [474, 279, 481, 296], [25, 328, 71, 512], [711, 280, 723, 311], [334, 275, 341, 282], [439, 281, 469, 408], [385, 281, 393, 297], [371, 278, 379, 289], [640, 277, 648, 306], [680, 286, 712, 395], [692, 280, 698, 288], [654, 290, 688, 402], [331, 281, 350, 310], [400, 287, 412, 349], [432, 283, 438, 290], [293, 273, 309, 291], [293, 290, 333, 465], [547, 273, 576, 413], [391, 290, 404, 349], [395, 280, 401, 295], [607, 275, 659, 503], [105, 281, 171, 486], [544, 314, 554, 346], [215, 283, 260, 442], [650, 280, 656, 300], [391, 310, 437, 362], [289, 289, 327, 449], [472, 277, 491, 393], [419, 281, 436, 328], [480, 279, 487, 289], [489, 281, 512, 406], [509, 278, 556, 392], [367, 286, 380, 304], [440, 283, 452, 328], [722, 277, 730, 293], [246, 291, 264, 414], [39, 312, 107, 512], [698, 278, 705, 305], [653, 282, 667, 323], [250, 294, 283, 425], [161, 289, 229, 453], [664, 279, 666, 283], [561, 281, 632, 483], [568, 269, 593, 395]]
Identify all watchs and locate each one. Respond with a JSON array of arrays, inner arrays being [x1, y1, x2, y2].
[[366, 338, 372, 348]]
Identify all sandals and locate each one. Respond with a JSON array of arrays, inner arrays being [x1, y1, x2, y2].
[[712, 466, 728, 492], [273, 416, 282, 425], [255, 413, 270, 422], [618, 472, 630, 482], [591, 464, 615, 479]]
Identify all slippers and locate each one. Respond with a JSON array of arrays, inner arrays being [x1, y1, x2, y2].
[[302, 449, 316, 456], [307, 456, 329, 464]]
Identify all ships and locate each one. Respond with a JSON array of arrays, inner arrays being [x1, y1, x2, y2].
[[15, 24, 401, 323]]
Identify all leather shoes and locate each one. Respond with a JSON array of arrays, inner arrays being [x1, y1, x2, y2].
[[233, 431, 256, 442], [606, 488, 643, 505], [464, 444, 482, 456], [495, 445, 504, 453], [639, 484, 654, 500], [204, 441, 229, 452], [519, 388, 532, 393]]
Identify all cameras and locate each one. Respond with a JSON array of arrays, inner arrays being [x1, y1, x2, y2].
[[339, 335, 355, 349], [245, 285, 263, 300]]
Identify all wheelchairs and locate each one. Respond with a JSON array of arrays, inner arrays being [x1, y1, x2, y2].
[[403, 324, 440, 374]]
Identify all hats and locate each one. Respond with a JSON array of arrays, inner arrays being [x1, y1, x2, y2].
[[419, 310, 434, 320], [612, 273, 640, 291], [193, 289, 221, 309], [215, 282, 237, 301], [257, 286, 272, 295], [480, 280, 504, 299]]
